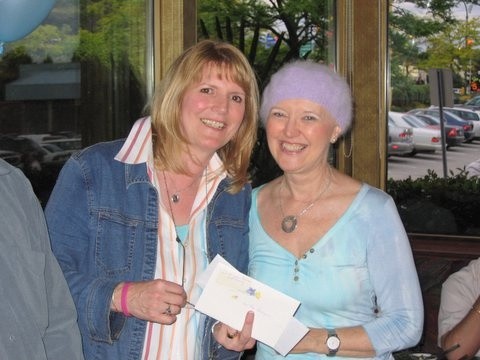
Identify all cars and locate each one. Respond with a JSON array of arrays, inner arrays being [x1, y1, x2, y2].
[[387, 96, 480, 157], [1, 133, 81, 188]]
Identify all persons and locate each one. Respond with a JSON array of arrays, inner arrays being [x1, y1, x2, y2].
[[0, 156, 84, 360], [248, 61, 424, 360], [41, 40, 261, 360], [437, 253, 480, 360]]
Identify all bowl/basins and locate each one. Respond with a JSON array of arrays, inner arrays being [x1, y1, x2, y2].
[[392, 346, 444, 360]]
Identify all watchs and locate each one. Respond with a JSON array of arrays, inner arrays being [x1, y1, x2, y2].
[[325, 327, 340, 357]]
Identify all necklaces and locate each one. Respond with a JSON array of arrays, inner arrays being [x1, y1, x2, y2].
[[154, 139, 209, 203], [278, 162, 332, 233]]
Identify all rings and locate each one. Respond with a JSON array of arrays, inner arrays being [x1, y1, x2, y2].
[[165, 304, 174, 315], [226, 328, 238, 339]]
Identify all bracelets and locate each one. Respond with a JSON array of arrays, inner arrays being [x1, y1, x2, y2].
[[111, 289, 120, 313], [121, 281, 133, 317]]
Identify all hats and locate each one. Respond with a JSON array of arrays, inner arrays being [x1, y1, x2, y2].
[[258, 61, 354, 133]]
